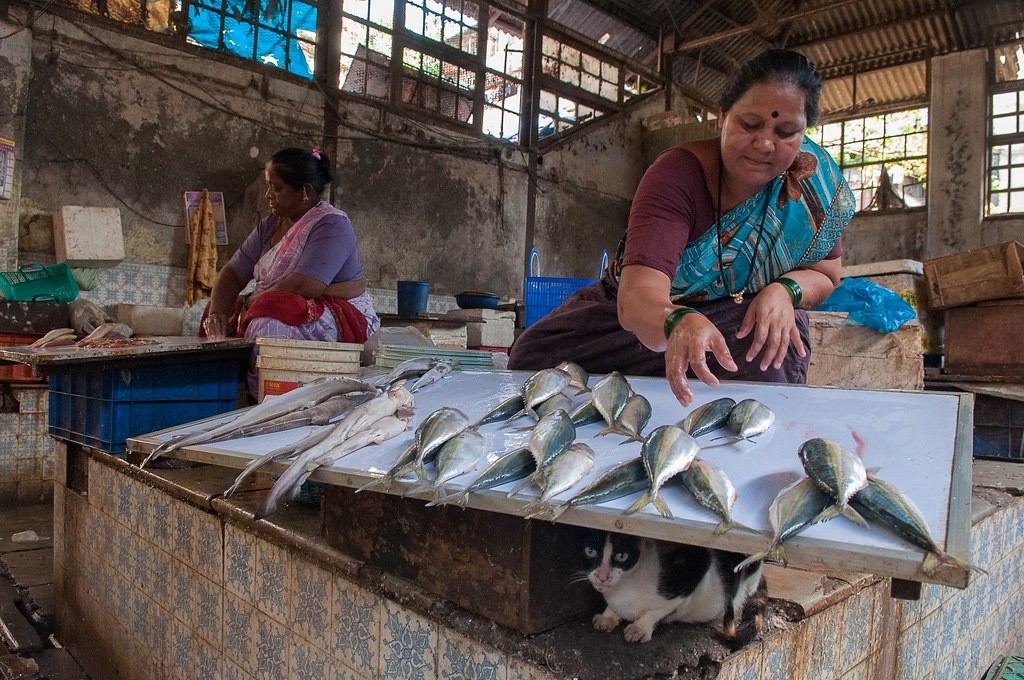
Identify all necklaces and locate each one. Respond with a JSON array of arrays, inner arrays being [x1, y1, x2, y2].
[[716, 153, 772, 305]]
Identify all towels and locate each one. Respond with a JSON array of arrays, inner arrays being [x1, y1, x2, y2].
[[186, 185, 219, 307]]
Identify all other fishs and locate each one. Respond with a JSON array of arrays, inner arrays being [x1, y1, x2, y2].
[[0, 320, 990, 583]]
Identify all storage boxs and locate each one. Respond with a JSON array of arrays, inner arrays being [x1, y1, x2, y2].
[[118, 303, 183, 336], [841, 259, 945, 354], [925, 386, 1024, 464], [804, 311, 924, 391], [448, 308, 516, 353], [924, 240, 1024, 376], [0, 333, 49, 382], [320, 482, 606, 636], [52, 205, 125, 268], [48, 360, 240, 454], [381, 318, 468, 349]]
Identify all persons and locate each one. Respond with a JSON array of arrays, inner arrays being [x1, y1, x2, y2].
[[197, 147, 380, 402], [507, 48, 856, 407]]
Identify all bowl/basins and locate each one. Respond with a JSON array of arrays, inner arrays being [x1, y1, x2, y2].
[[454, 294, 500, 309]]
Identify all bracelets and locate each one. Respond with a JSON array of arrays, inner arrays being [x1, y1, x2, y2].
[[762, 277, 801, 308], [663, 306, 701, 339], [241, 297, 250, 309]]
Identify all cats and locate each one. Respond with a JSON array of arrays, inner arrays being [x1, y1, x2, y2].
[[578, 529, 762, 644]]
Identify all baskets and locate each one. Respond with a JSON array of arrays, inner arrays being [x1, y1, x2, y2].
[[0, 263, 79, 304], [525, 248, 609, 329]]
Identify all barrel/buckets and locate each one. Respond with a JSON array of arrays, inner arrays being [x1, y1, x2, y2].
[[396, 281, 431, 316]]
